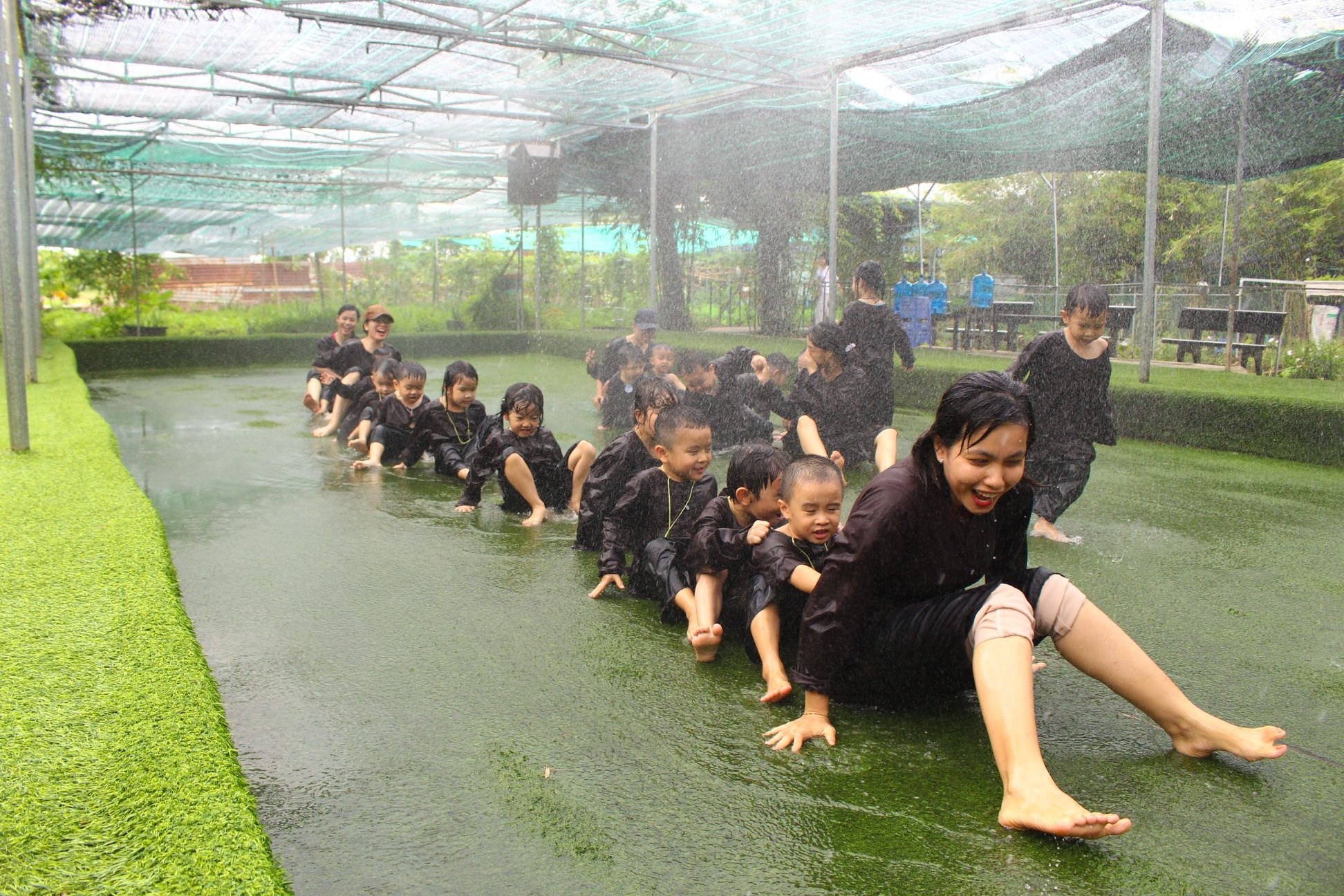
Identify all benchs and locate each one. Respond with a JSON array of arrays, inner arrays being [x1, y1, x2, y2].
[[946, 299, 1137, 358], [1162, 306, 1287, 374]]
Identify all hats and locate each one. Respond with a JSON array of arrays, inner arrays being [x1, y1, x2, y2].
[[633, 308, 660, 331], [364, 304, 394, 323]]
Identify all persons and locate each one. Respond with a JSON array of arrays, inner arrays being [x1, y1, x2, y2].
[[737, 348, 807, 440], [782, 322, 898, 488], [810, 253, 838, 326], [678, 346, 792, 451], [1002, 283, 1117, 542], [455, 383, 596, 526], [303, 305, 402, 437], [572, 378, 844, 704], [332, 357, 501, 480], [762, 372, 1288, 838], [839, 260, 916, 426], [586, 308, 686, 429]]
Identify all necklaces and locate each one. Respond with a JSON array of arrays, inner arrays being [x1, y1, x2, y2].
[[728, 496, 748, 520], [401, 398, 413, 415], [445, 398, 471, 444], [662, 465, 695, 537], [792, 537, 828, 569]]
